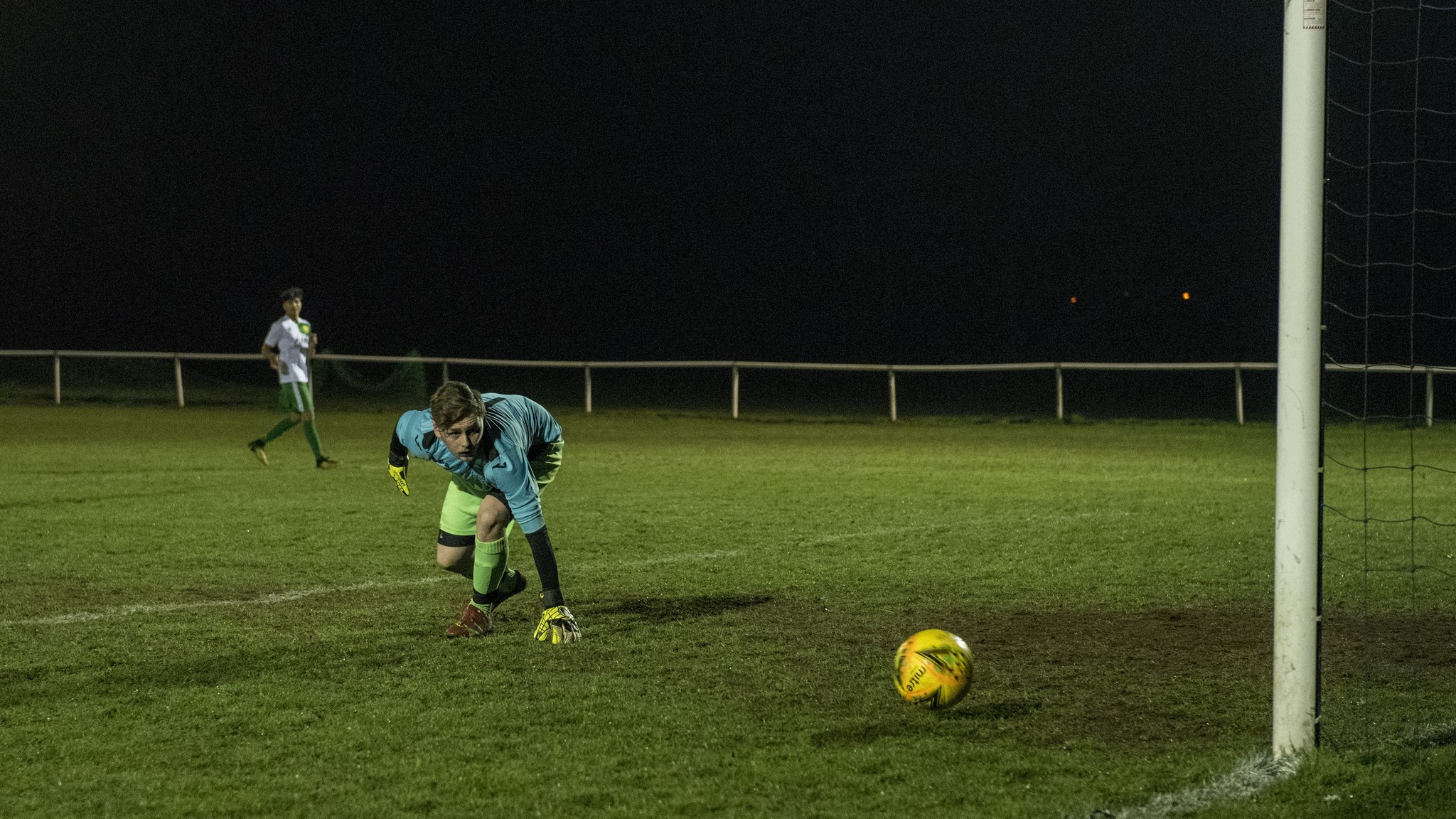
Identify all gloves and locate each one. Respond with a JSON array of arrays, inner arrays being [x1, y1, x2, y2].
[[534, 588, 582, 645], [387, 449, 411, 496]]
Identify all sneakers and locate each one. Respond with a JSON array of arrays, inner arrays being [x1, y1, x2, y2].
[[316, 454, 342, 469], [490, 570, 527, 613], [248, 441, 269, 467], [445, 601, 492, 638]]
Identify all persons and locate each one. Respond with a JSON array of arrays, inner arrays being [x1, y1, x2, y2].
[[388, 382, 585, 643], [249, 287, 343, 467]]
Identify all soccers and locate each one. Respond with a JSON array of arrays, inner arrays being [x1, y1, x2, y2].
[[893, 628, 974, 711]]
